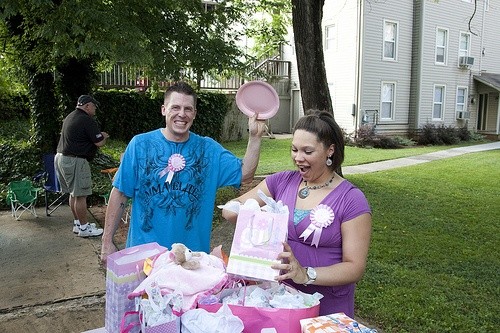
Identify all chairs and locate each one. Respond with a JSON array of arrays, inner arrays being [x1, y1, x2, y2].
[[101, 152, 124, 182], [33, 153, 70, 217], [5, 180, 46, 220], [97, 192, 132, 225]]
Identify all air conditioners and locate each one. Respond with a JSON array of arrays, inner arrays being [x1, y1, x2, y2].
[[456, 111, 471, 120], [459, 56, 475, 67]]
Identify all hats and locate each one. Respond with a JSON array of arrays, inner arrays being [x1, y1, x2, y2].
[[76, 95, 100, 106]]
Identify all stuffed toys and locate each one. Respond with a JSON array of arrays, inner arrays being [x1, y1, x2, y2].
[[169, 243, 201, 270]]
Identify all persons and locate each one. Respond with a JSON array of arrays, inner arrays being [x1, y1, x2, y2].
[[101, 81, 266, 269], [222, 109, 372, 321], [54, 94, 110, 237]]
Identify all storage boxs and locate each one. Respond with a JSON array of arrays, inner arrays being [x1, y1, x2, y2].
[[299, 313, 368, 333]]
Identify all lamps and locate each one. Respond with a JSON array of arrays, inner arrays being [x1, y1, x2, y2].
[[471, 95, 477, 105]]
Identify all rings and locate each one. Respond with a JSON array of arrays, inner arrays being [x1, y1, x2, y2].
[[288, 264, 293, 272]]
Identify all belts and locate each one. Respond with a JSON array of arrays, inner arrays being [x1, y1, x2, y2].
[[57, 152, 86, 159]]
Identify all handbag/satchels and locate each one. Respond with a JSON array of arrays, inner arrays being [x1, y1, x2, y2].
[[226, 207, 289, 285], [104, 242, 169, 333], [197, 279, 320, 333], [121, 298, 182, 333]]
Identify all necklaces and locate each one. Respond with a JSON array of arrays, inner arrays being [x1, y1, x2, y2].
[[298, 171, 336, 199]]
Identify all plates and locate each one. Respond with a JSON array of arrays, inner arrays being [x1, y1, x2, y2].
[[235, 81, 279, 120]]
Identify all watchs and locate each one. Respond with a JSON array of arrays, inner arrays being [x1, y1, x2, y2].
[[303, 266, 317, 286]]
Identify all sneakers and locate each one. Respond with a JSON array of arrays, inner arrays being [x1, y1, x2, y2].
[[77, 225, 103, 238], [73, 221, 96, 234]]
